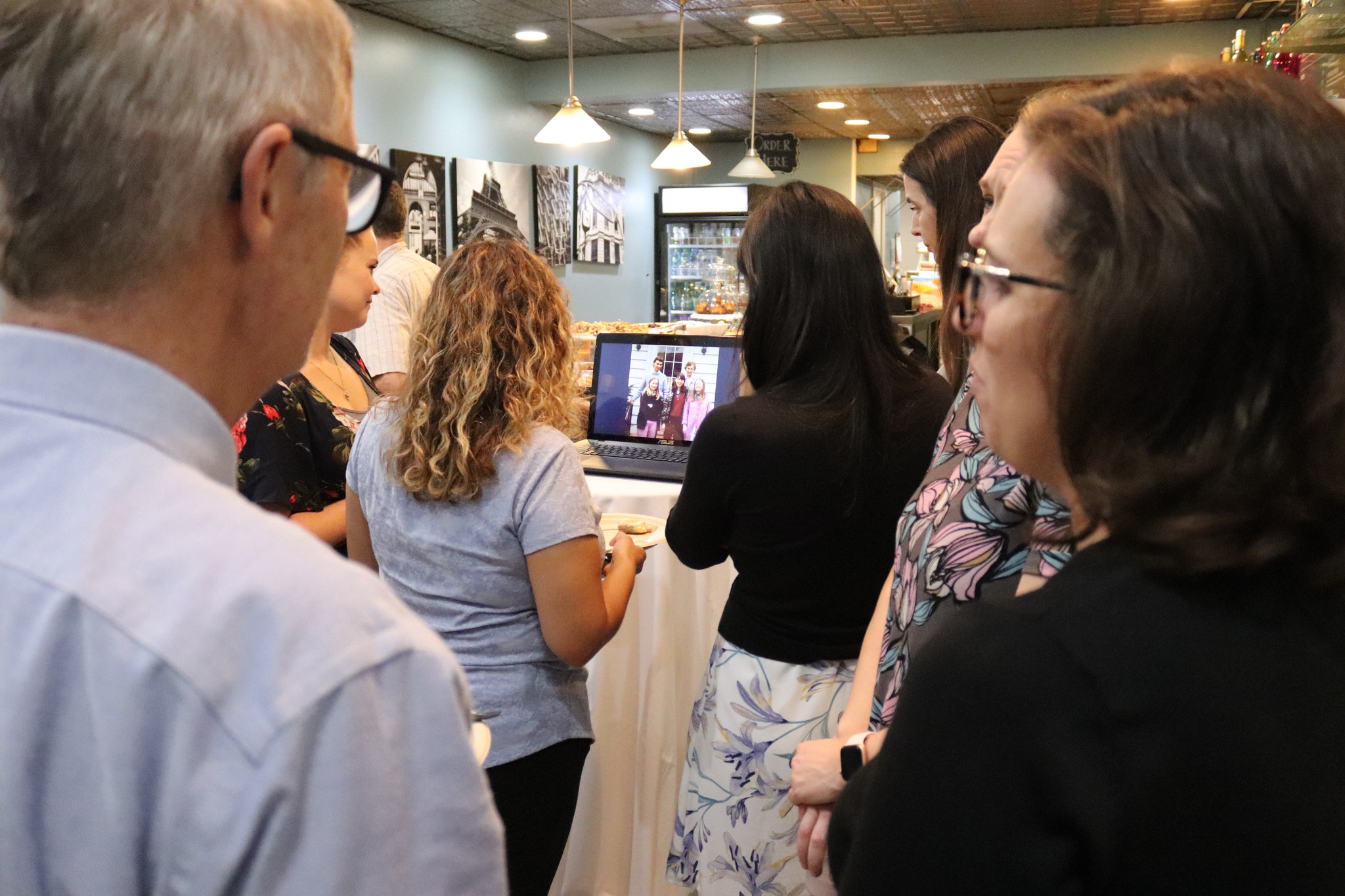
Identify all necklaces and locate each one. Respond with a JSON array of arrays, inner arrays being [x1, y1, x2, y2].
[[304, 350, 352, 402]]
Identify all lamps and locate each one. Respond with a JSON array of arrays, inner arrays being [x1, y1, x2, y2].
[[727, 36, 776, 178], [650, 0, 711, 169], [533, 0, 611, 147]]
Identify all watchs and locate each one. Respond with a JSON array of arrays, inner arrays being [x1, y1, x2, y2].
[[838, 731, 877, 781]]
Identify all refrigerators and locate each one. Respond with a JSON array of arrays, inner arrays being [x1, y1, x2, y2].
[[653, 182, 764, 323]]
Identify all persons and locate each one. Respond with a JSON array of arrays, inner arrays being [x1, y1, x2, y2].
[[344, 233, 647, 896], [625, 354, 713, 442], [824, 70, 1344, 896], [227, 226, 382, 557], [657, 185, 953, 896], [788, 116, 1079, 896], [2, 1, 511, 896], [333, 179, 444, 401]]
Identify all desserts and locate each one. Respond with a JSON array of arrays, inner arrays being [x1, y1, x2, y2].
[[618, 519, 655, 534], [696, 301, 735, 314]]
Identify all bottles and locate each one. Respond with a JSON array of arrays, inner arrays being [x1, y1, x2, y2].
[[671, 247, 717, 276], [672, 226, 745, 244], [1220, 23, 1299, 80], [669, 282, 710, 310]]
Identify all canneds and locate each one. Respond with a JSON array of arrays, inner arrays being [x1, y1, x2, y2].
[[722, 227, 746, 244]]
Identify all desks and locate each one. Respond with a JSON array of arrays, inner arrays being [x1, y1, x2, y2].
[[549, 472, 737, 896], [889, 306, 942, 372]]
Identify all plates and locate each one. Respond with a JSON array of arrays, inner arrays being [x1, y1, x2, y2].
[[465, 710, 492, 766], [685, 323, 729, 336], [599, 513, 667, 553], [569, 362, 594, 376]]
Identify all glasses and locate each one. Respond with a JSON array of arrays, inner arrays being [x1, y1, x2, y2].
[[957, 260, 1074, 329], [230, 126, 397, 234]]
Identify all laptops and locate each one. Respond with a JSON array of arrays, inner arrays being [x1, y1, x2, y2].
[[573, 333, 743, 479]]
